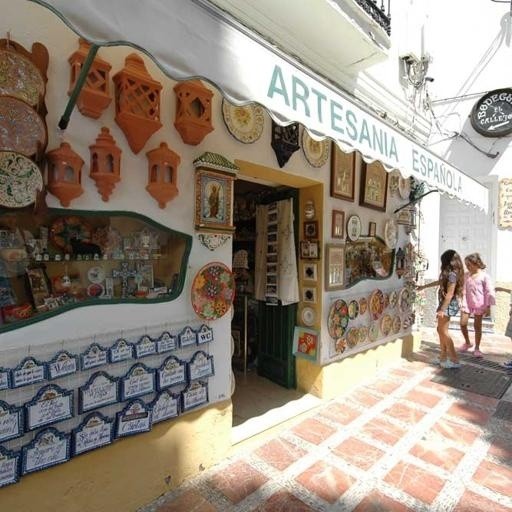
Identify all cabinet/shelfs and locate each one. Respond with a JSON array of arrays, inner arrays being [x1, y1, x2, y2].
[[1, 243, 187, 339]]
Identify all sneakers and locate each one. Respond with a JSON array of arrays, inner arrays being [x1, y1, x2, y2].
[[499, 359, 512, 369]]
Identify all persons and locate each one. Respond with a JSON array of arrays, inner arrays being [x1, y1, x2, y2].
[[459, 252, 497, 359], [495, 286, 512, 370], [417, 249, 466, 369]]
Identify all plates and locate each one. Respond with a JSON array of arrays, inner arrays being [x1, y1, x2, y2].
[[327, 289, 416, 357], [398, 176, 412, 200], [346, 214, 362, 242], [301, 127, 329, 169], [385, 218, 400, 249], [300, 308, 317, 327], [222, 95, 264, 143], [0, 51, 45, 207]]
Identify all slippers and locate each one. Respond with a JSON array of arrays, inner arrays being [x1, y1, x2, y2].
[[459, 343, 473, 353], [471, 350, 482, 358], [429, 357, 441, 365], [440, 359, 461, 369]]
